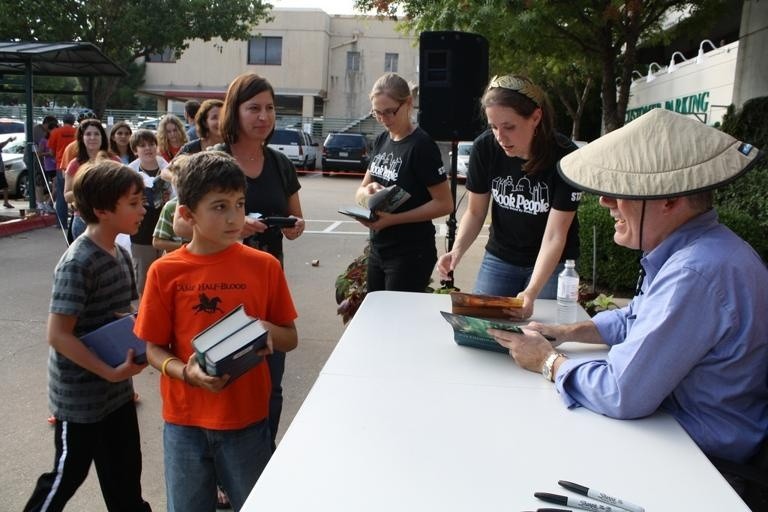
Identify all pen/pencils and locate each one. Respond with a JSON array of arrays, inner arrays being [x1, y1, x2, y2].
[[534, 492, 611, 512], [558, 480, 625, 509], [537, 508, 573, 512]]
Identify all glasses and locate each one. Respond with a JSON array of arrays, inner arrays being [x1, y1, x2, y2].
[[369, 102, 405, 118]]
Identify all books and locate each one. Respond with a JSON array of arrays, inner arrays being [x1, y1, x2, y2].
[[203, 319, 268, 390], [451, 292, 524, 322], [440, 312, 555, 356], [75, 311, 147, 380], [191, 303, 259, 374], [338, 184, 411, 222]]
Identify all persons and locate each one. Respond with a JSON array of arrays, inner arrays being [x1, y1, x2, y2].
[[47, 113, 76, 228], [22, 161, 150, 512], [435, 75, 580, 319], [1, 137, 17, 208], [356, 72, 453, 294], [111, 123, 139, 165], [39, 121, 58, 215], [159, 115, 187, 164], [124, 130, 176, 317], [488, 107, 767, 511], [153, 153, 191, 254], [23, 115, 58, 209], [204, 73, 305, 453], [152, 99, 223, 208], [183, 99, 200, 142], [64, 119, 121, 247], [59, 109, 97, 177], [133, 152, 298, 510]]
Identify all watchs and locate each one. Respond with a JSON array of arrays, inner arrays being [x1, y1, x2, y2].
[[541, 352, 568, 382]]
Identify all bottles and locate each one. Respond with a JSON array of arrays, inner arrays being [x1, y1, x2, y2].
[[557, 259, 579, 325]]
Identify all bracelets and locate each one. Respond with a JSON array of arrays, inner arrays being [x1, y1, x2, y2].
[[162, 356, 181, 378]]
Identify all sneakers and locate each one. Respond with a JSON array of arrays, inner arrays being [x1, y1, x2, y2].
[[45, 202, 57, 215], [37, 206, 48, 216]]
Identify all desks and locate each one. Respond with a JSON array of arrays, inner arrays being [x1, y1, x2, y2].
[[240, 289, 752, 512]]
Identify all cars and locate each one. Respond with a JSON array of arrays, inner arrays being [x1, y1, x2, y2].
[[0, 133, 29, 198], [449, 142, 473, 184]]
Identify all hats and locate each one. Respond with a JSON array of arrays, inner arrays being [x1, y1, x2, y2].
[[557, 106, 763, 201]]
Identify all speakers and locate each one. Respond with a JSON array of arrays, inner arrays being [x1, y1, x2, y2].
[[418, 31, 489, 142]]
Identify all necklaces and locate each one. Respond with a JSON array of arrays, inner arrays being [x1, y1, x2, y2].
[[238, 141, 263, 161]]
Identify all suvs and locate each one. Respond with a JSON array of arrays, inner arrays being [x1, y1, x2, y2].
[[321, 130, 373, 177], [262, 128, 319, 175]]
